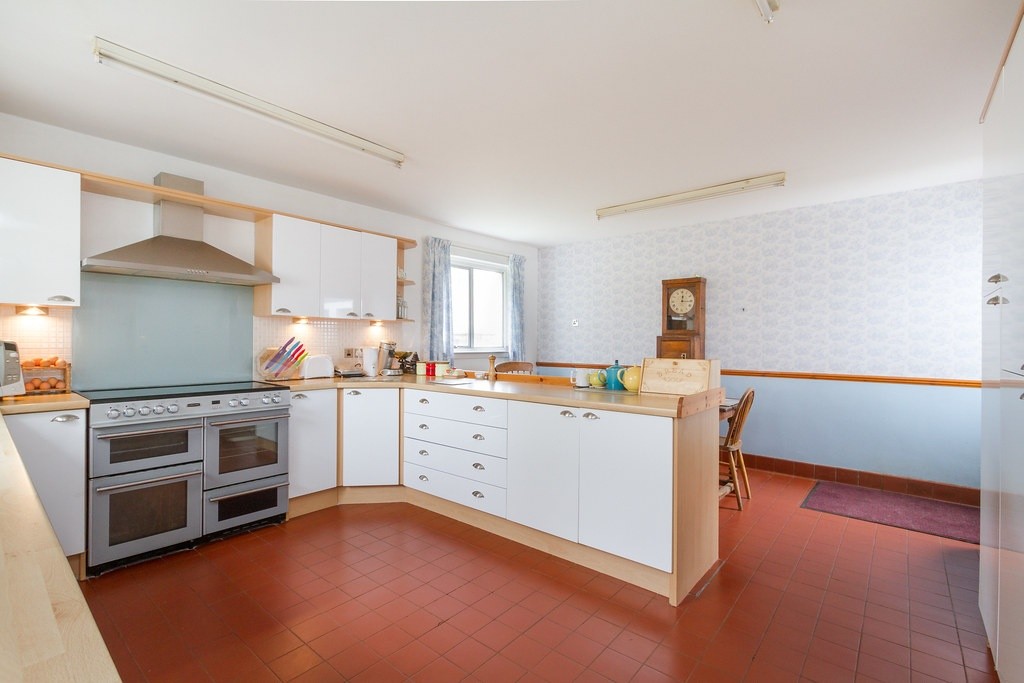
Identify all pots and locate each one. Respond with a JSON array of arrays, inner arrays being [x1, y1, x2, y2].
[[336, 368, 364, 376]]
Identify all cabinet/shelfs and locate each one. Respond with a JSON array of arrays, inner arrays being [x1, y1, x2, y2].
[[4, 408, 86, 559], [20, 364, 73, 395], [289, 391, 338, 497], [506, 401, 719, 607], [342, 388, 399, 486], [0, 157, 82, 308], [403, 389, 507, 519], [978, 0, 1024, 682], [254, 214, 419, 323]]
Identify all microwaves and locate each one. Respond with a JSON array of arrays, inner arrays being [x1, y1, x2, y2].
[[0, 341, 26, 397]]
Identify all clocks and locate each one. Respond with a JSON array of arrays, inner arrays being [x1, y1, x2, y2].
[[656, 278, 707, 359]]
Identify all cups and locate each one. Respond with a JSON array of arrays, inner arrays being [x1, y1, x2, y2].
[[570, 368, 590, 386], [426, 363, 435, 376], [597, 359, 626, 390]]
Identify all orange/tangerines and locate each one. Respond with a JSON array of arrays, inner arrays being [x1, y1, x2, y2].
[[21, 356, 66, 368], [25, 377, 66, 390]]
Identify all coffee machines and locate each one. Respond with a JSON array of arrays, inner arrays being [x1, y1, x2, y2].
[[379, 340, 403, 375]]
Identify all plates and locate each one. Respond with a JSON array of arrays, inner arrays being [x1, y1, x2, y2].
[[443, 374, 467, 379]]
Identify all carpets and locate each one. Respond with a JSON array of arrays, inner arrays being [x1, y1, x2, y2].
[[801, 482, 980, 545]]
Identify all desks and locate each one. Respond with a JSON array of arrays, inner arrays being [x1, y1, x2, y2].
[[720, 397, 739, 501]]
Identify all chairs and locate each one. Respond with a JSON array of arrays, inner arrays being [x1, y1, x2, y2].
[[495, 362, 534, 374], [719, 387, 755, 512]]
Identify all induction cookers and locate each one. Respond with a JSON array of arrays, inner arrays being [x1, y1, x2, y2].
[[73, 380, 291, 429]]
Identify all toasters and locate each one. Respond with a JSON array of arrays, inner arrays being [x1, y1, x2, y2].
[[299, 354, 335, 379]]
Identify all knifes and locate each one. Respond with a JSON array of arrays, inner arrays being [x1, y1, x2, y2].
[[264, 336, 310, 380]]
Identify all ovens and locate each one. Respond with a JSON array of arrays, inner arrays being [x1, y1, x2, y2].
[[87, 408, 289, 576]]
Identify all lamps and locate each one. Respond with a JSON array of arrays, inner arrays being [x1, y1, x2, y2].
[[16, 306, 49, 315], [91, 35, 404, 169], [754, 0, 780, 24], [291, 317, 308, 324], [596, 172, 785, 221], [369, 321, 385, 326]]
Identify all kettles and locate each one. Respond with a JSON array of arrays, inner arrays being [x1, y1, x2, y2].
[[363, 345, 378, 377]]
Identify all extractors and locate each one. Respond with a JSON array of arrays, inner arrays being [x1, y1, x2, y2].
[[79, 173, 281, 287]]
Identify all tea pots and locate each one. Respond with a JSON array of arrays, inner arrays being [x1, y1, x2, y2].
[[617, 363, 642, 392], [587, 370, 606, 387]]
